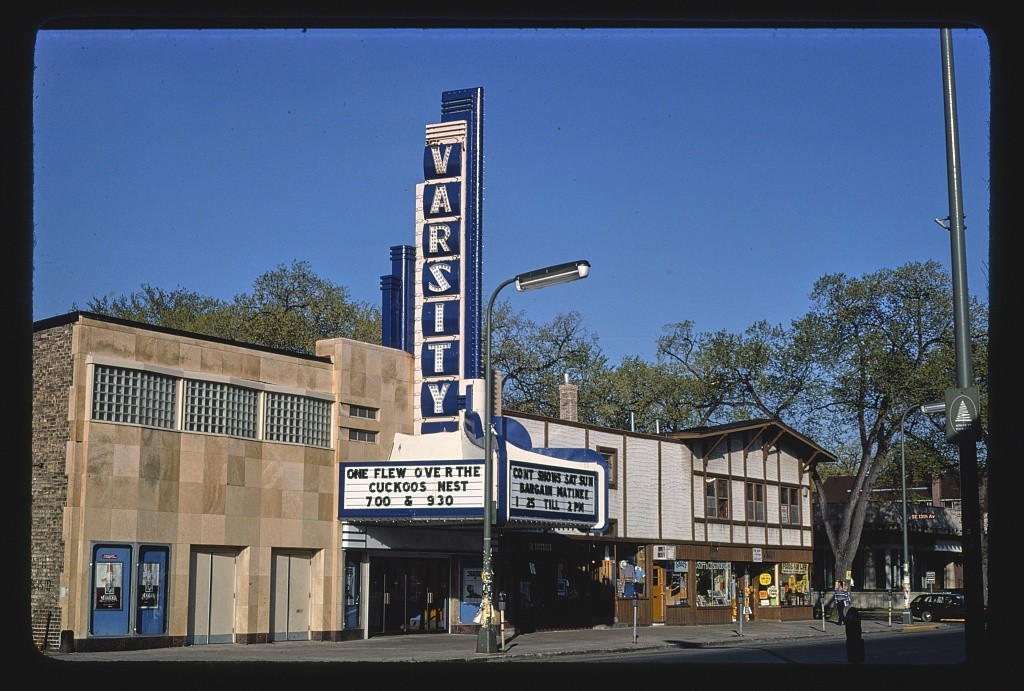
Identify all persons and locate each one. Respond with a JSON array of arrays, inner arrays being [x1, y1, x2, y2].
[[466, 585, 482, 598], [345, 584, 353, 600], [833, 581, 849, 625]]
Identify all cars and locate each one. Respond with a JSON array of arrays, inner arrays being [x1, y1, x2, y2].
[[910, 591, 966, 624]]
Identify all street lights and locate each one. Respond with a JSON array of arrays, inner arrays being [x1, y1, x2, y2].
[[896, 399, 952, 618], [467, 257, 592, 654]]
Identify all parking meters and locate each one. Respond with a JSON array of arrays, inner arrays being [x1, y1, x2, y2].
[[630, 592, 640, 644], [499, 591, 507, 652], [819, 590, 827, 633], [737, 587, 744, 637]]
